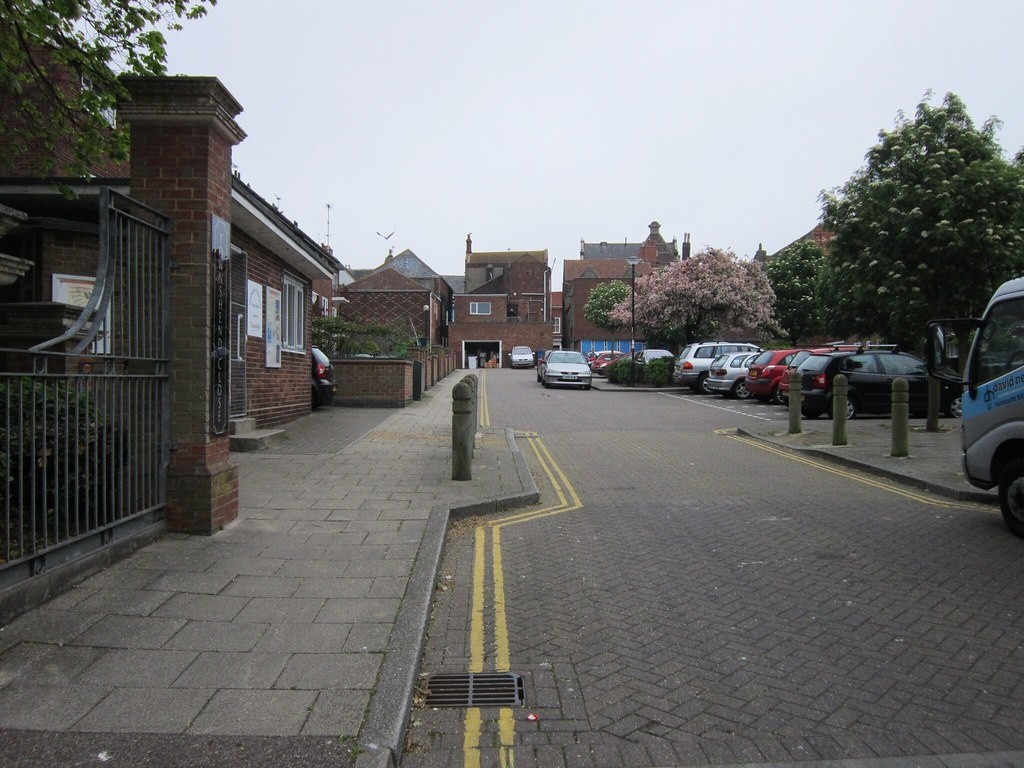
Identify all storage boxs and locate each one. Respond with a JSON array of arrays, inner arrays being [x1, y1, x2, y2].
[[484, 358, 499, 368]]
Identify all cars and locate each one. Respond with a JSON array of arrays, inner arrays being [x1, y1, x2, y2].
[[585, 349, 676, 377], [537, 350, 593, 391], [744, 347, 864, 409], [706, 352, 762, 399], [508, 346, 536, 370]]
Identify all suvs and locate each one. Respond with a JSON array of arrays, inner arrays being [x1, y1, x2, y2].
[[796, 344, 965, 420], [310, 345, 339, 412], [673, 341, 766, 395]]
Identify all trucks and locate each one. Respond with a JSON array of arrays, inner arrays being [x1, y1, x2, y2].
[[922, 276, 1024, 543]]
